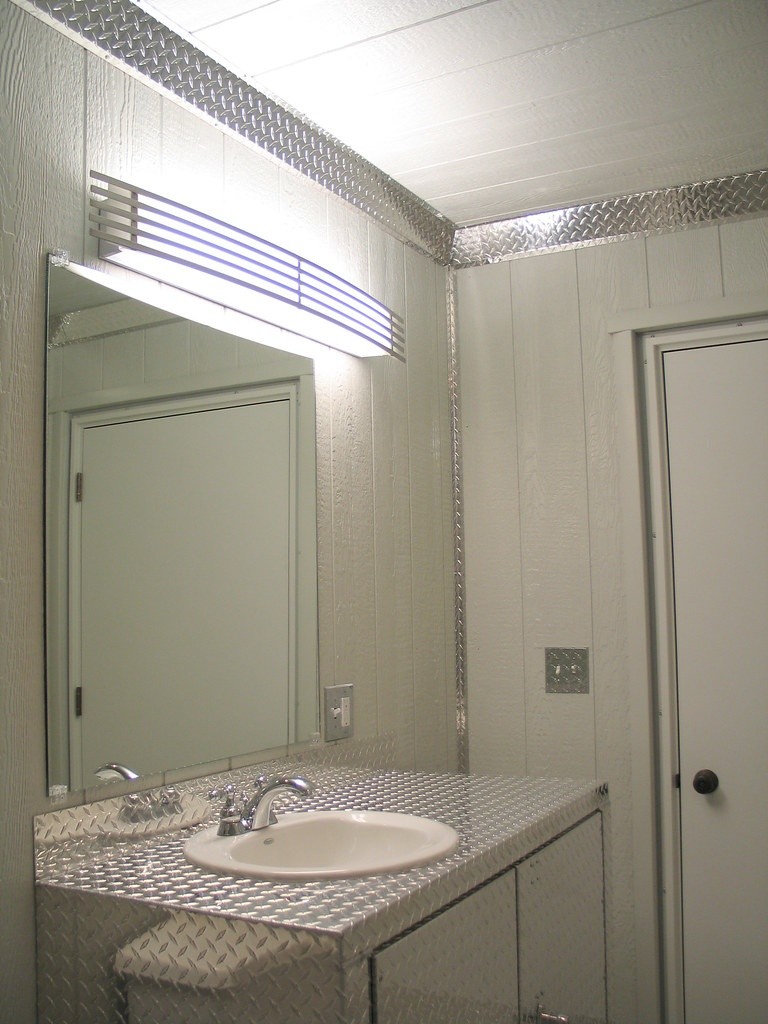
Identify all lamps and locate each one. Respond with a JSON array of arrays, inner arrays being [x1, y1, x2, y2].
[[87, 171, 408, 365]]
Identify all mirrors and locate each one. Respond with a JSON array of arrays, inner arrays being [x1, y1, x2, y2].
[[47, 253, 319, 799]]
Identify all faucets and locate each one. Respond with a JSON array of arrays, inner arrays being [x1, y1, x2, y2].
[[241, 778, 317, 829], [93, 763, 137, 780]]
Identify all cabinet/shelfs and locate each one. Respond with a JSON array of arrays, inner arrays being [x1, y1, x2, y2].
[[343, 781, 611, 1024]]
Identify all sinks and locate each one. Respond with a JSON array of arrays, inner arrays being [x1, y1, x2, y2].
[[184, 811, 460, 878]]
